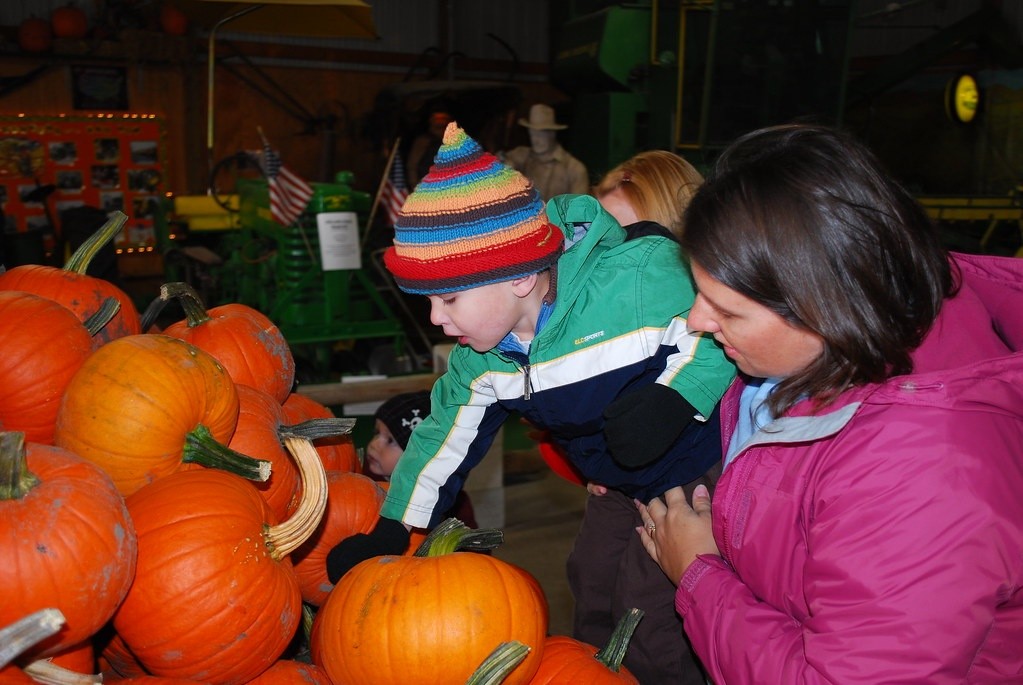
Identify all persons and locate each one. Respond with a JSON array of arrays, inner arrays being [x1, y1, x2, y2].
[[505, 106, 590, 202], [326, 121, 739, 685], [363, 392, 490, 554], [634, 124, 1023, 685], [596, 152, 704, 230]]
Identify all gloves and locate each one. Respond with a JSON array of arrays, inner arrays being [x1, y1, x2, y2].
[[326, 516, 410, 586]]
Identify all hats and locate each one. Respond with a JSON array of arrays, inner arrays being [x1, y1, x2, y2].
[[519, 104, 568, 129], [372, 390, 431, 451], [383, 121, 565, 294]]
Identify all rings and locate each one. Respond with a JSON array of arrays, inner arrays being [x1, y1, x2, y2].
[[650, 526, 656, 537]]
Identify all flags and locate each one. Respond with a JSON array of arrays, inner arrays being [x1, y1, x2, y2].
[[265, 146, 313, 226], [380, 152, 410, 224]]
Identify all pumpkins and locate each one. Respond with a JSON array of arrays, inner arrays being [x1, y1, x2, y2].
[[1, 264, 640, 685]]
